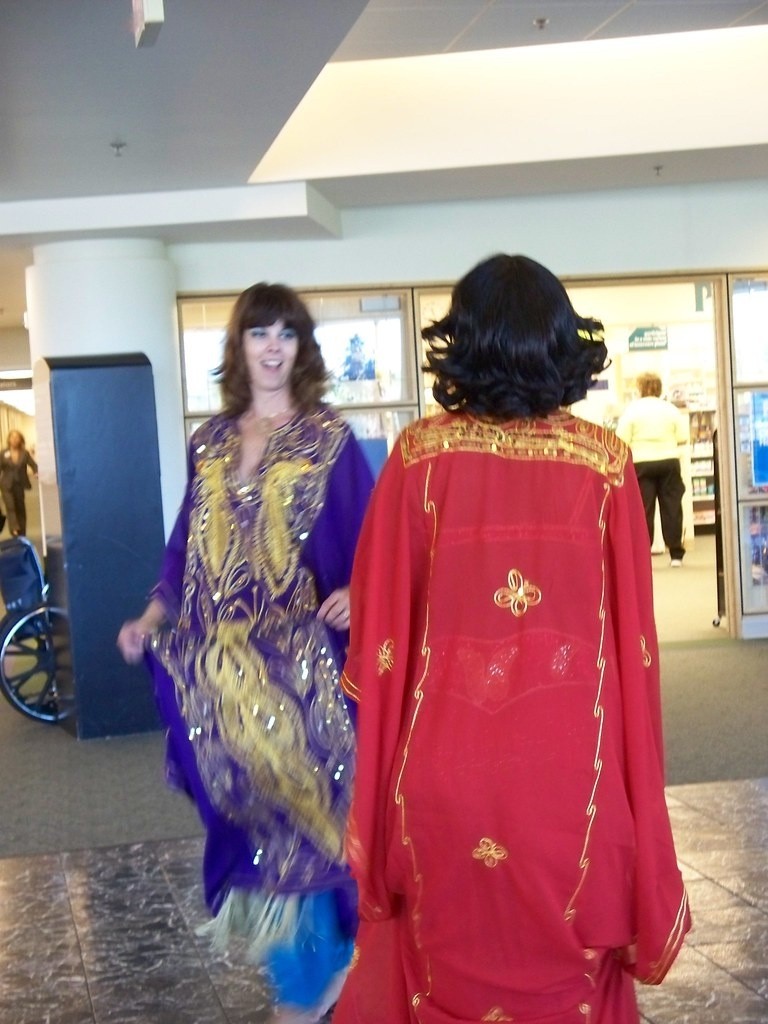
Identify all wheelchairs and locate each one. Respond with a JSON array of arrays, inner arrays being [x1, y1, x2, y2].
[[1, 535, 77, 727]]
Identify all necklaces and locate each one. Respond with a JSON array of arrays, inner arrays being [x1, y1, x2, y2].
[[252, 407, 289, 434]]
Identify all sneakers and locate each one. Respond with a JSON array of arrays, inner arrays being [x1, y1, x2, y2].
[[668, 559, 683, 568]]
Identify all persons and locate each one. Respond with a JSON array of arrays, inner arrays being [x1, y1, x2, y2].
[[615, 374, 689, 567], [117, 282, 374, 1024], [0, 429, 38, 537], [336, 254, 691, 1024]]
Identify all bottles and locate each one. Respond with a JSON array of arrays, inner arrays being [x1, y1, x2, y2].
[[689, 412, 708, 443]]
